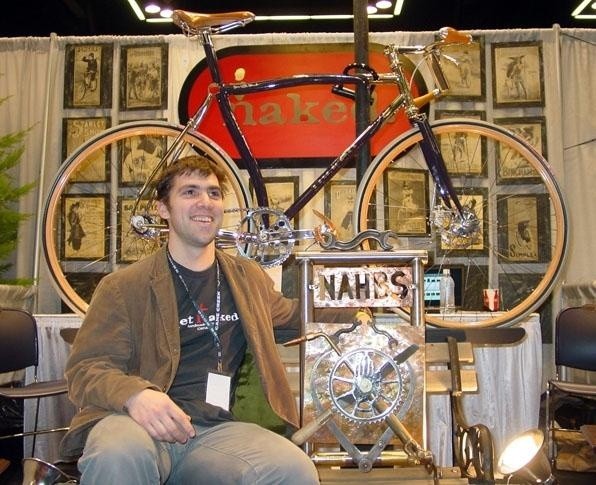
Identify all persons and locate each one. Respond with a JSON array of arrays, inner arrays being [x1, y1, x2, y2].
[[67, 52, 159, 251], [454, 48, 534, 259], [66, 156, 365, 485]]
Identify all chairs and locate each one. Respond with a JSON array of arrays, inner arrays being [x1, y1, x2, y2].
[[0, 306, 81, 458], [545, 302, 596, 476]]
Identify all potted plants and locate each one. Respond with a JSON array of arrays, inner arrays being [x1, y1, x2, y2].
[[0, 69, 43, 386]]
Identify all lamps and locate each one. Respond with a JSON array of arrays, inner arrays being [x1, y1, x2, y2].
[[495, 429, 557, 484]]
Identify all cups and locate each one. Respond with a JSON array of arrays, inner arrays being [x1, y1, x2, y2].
[[483, 289, 501, 312]]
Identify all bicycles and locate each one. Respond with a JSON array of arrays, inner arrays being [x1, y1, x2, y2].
[[459, 61, 472, 88], [74, 71, 98, 103], [42, 8, 572, 336]]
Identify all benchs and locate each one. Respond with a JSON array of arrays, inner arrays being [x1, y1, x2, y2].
[[59, 325, 494, 484]]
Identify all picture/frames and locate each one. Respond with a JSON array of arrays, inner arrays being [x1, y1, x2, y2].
[[431, 33, 552, 265], [60, 42, 171, 266], [382, 167, 432, 238], [322, 179, 378, 250], [248, 175, 300, 247]]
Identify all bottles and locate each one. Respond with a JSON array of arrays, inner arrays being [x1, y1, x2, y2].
[[439, 269, 456, 315]]
